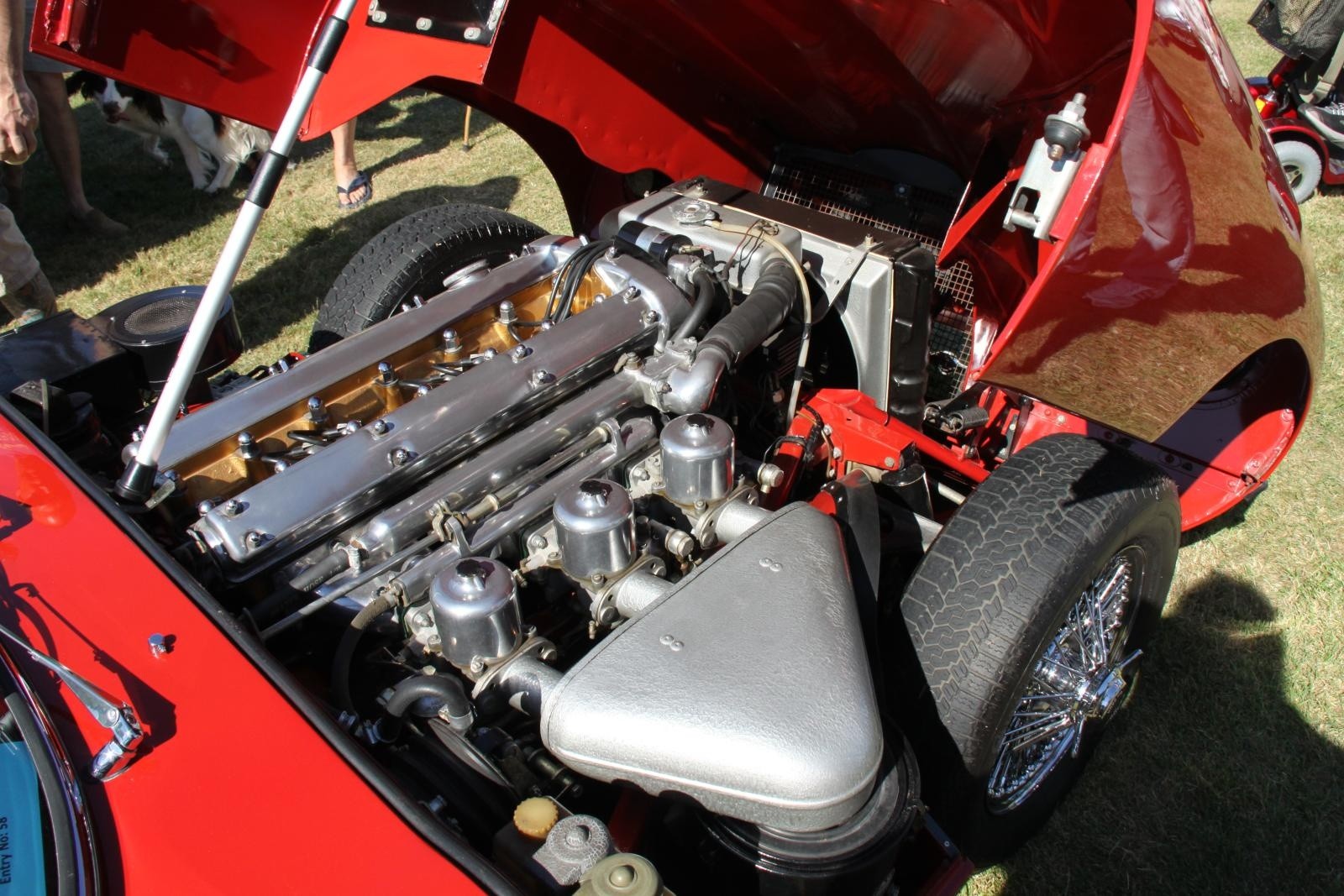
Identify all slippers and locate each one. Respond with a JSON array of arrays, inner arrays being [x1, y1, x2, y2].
[[336, 170, 371, 208]]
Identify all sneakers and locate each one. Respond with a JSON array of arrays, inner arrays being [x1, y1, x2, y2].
[[1297, 102, 1344, 150]]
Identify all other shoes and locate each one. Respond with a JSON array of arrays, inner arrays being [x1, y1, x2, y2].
[[67, 209, 128, 238], [3, 173, 25, 219]]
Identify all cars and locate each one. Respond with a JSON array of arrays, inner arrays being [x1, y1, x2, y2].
[[0, 0, 1344, 894]]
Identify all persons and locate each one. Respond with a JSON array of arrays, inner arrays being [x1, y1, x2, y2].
[[0, 0, 135, 327], [1059, 52, 1200, 309], [329, 116, 371, 210]]
